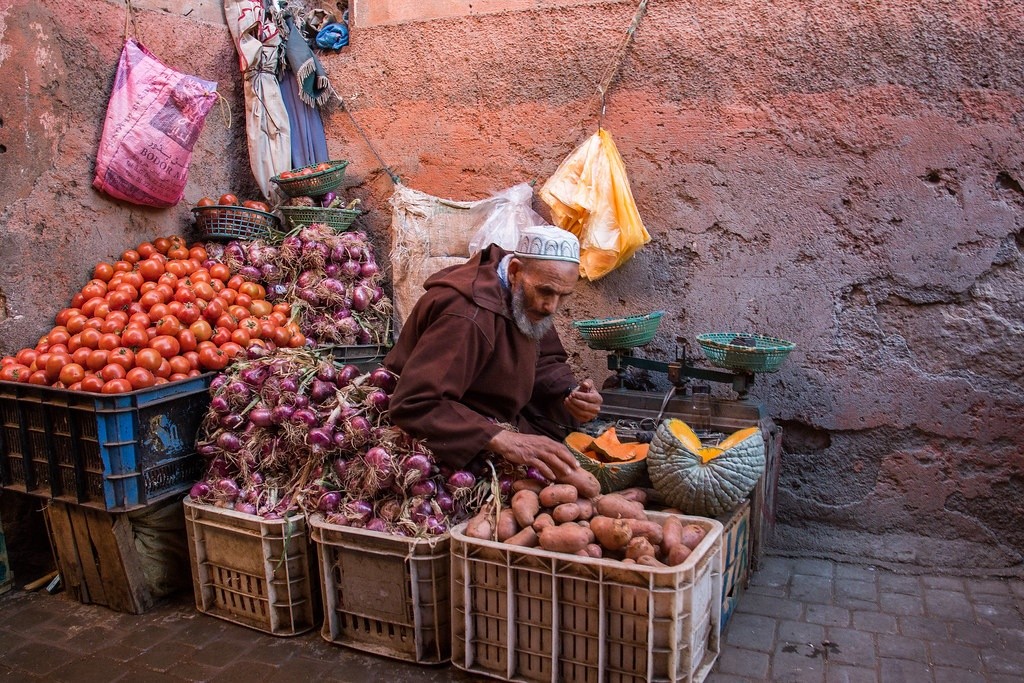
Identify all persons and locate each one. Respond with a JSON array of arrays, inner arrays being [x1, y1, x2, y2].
[[380, 223, 604, 479]]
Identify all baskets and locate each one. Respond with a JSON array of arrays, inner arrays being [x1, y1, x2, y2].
[[573, 312, 664, 349], [279, 206, 361, 231], [191, 206, 281, 240], [697, 333, 796, 372], [270, 160, 348, 197]]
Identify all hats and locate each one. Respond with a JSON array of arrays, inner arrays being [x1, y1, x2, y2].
[[515, 226, 580, 264]]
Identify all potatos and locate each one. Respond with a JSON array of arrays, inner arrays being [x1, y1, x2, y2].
[[464, 464, 707, 571]]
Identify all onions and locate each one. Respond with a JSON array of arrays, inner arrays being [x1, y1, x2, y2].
[[283, 190, 337, 207], [183, 223, 554, 539]]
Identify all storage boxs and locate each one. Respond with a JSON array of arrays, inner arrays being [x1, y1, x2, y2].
[[0, 338, 722, 683]]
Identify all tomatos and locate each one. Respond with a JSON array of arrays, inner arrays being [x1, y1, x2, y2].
[[279, 162, 334, 193], [0, 235, 307, 394], [196, 194, 269, 235]]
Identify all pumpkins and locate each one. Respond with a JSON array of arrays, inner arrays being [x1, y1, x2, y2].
[[645, 418, 766, 515], [563, 427, 651, 494]]
